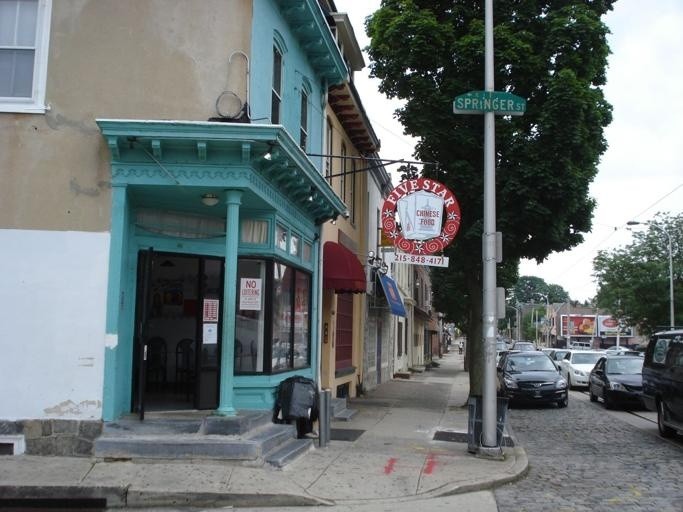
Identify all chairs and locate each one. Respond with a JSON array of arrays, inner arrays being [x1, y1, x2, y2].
[[234, 340, 242, 374], [250, 339, 257, 370], [175, 338, 197, 402], [146, 337, 168, 393]]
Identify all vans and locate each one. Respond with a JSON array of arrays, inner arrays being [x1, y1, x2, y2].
[[642, 330, 683, 438]]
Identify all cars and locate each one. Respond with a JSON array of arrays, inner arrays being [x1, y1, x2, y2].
[[499, 351, 568, 408], [496, 334, 644, 366], [589, 356, 645, 408], [558, 349, 608, 388]]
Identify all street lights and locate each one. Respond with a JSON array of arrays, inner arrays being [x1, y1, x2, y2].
[[626, 221, 675, 330]]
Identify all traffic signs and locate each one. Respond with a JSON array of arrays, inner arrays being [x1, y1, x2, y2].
[[455, 90, 527, 115]]
